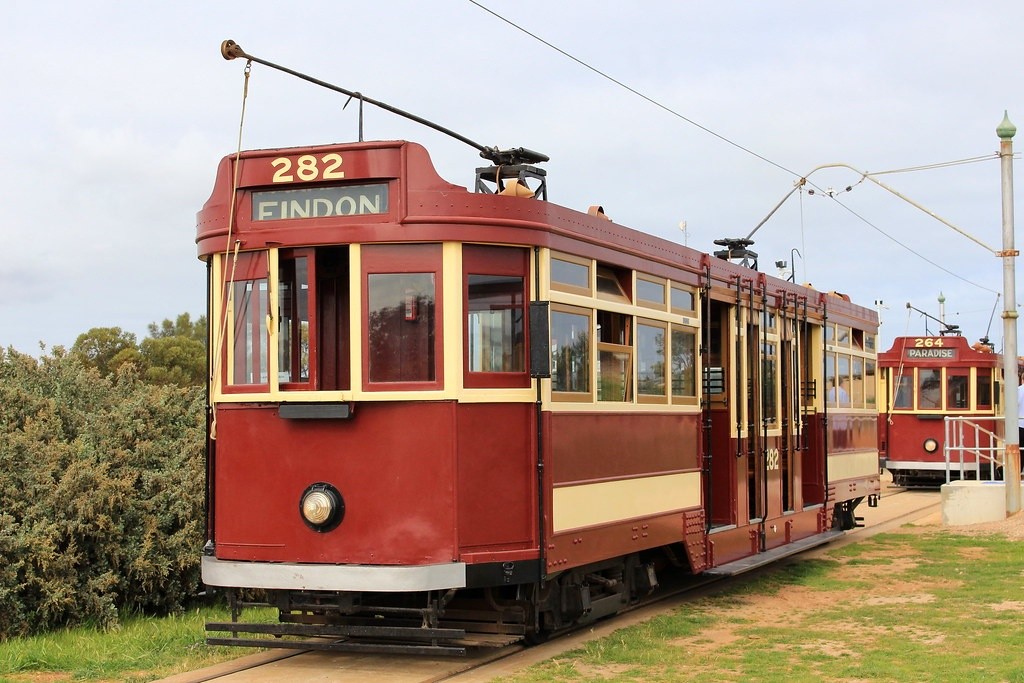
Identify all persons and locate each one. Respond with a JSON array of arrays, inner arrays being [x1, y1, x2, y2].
[[828, 376, 849, 450], [1018, 372, 1024, 480]]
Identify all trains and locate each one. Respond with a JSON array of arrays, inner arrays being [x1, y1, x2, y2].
[[193, 38, 892, 659], [878, 301, 1024, 490]]
